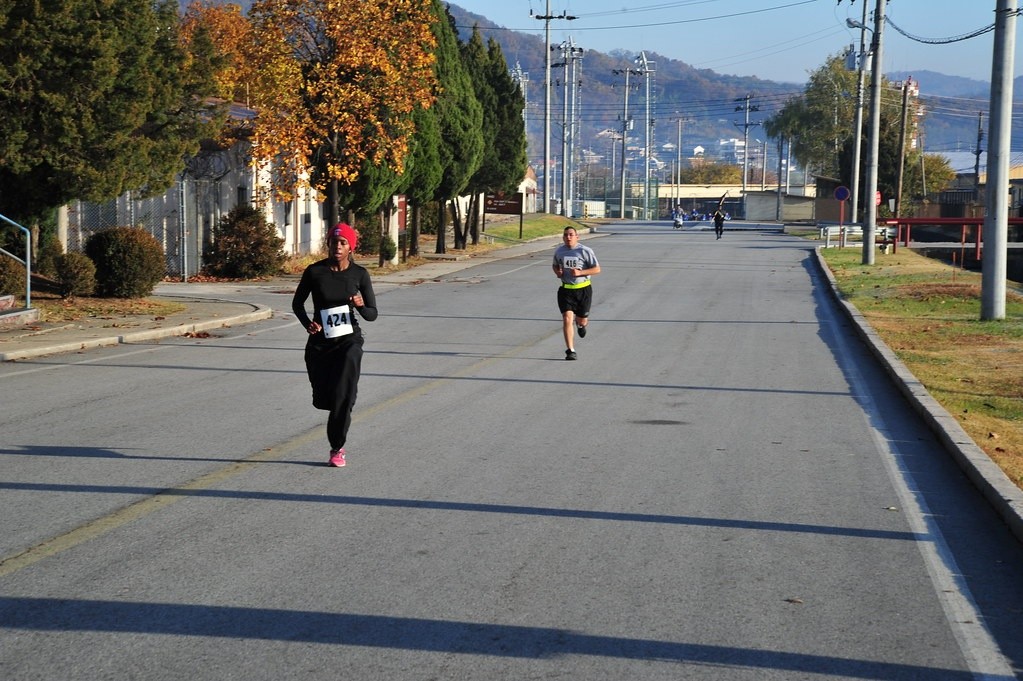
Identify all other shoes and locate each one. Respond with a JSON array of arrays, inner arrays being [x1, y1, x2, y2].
[[716, 237, 721, 240]]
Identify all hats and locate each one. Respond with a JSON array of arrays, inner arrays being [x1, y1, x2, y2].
[[327, 223, 357, 254]]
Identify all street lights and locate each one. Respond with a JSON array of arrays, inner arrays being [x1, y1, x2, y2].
[[844, 16, 882, 265]]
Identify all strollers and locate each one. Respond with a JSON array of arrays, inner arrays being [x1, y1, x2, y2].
[[674, 212, 683, 229]]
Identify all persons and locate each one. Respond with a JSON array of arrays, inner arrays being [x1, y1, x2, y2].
[[701, 212, 731, 221], [292, 224, 379, 467], [713, 205, 725, 240], [552, 227, 600, 359], [671, 205, 699, 221]]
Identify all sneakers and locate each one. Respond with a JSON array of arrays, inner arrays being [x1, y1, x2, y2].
[[329, 449, 347, 467], [565, 348, 577, 360], [576, 322, 586, 338]]
